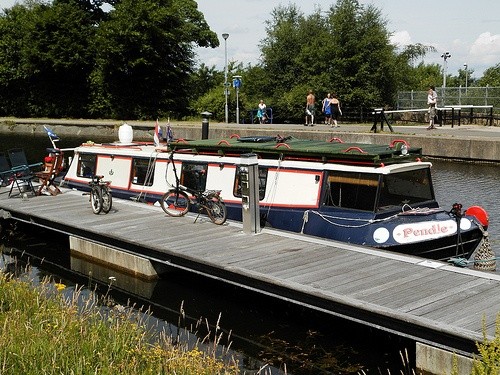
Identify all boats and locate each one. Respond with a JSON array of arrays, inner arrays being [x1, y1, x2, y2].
[[49, 133, 487, 257]]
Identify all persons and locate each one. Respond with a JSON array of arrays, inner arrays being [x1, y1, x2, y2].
[[305, 90, 315, 126], [427, 85, 438, 130], [322, 93, 333, 125], [257, 100, 267, 124], [325, 93, 342, 128]]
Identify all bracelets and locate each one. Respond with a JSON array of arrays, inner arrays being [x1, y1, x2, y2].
[[322, 108, 324, 109]]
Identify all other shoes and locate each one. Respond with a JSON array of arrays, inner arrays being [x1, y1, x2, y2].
[[311, 125, 313, 126], [304, 123, 308, 126]]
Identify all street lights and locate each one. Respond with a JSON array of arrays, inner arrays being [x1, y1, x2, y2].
[[221, 33, 230, 123]]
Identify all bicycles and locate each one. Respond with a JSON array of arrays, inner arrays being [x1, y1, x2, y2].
[[88, 175, 112, 215], [160, 150, 227, 226]]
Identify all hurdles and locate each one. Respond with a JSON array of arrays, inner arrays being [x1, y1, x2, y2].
[[369, 104, 495, 133]]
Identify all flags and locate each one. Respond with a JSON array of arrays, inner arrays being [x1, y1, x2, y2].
[[44, 126, 62, 141], [154, 121, 163, 146], [166, 123, 175, 143]]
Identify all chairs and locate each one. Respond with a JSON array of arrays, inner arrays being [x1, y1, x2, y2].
[[1, 146, 64, 199]]
